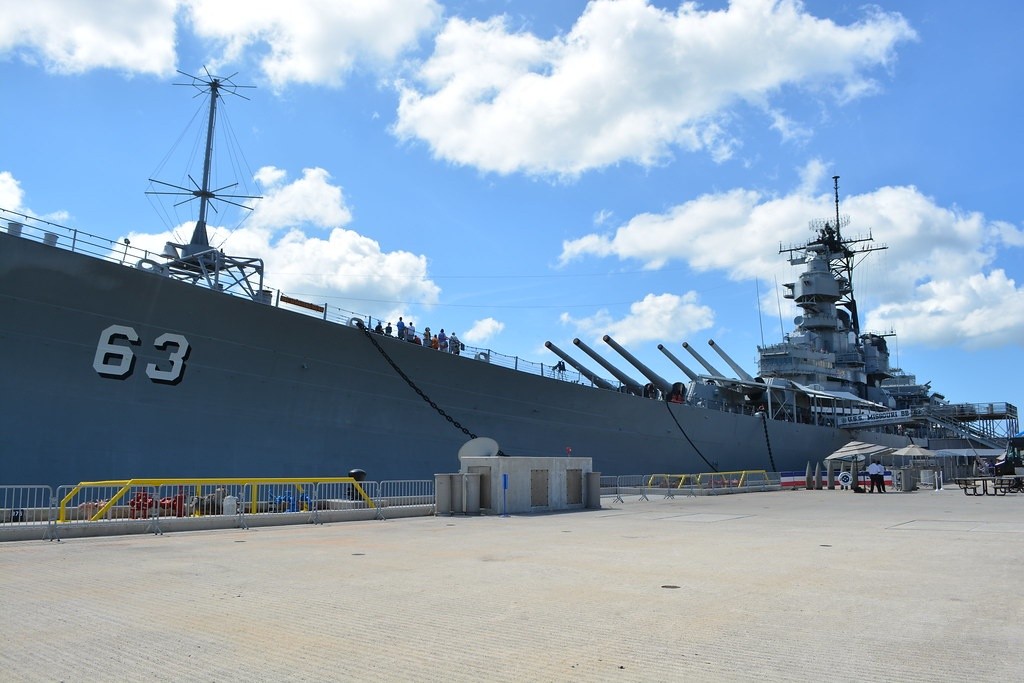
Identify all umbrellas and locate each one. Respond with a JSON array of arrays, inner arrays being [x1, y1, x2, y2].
[[825, 439, 898, 486], [876, 443, 962, 483]]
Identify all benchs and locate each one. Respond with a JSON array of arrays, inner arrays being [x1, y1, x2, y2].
[[955, 477, 1015, 496]]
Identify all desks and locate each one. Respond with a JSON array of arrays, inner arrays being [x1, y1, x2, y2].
[[1003, 475, 1024, 493], [969, 478, 996, 496]]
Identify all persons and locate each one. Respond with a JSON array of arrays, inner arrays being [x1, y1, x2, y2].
[[345, 468, 369, 501], [868, 459, 883, 493], [397, 316, 405, 338], [974, 456, 1016, 479], [404, 321, 431, 348], [449, 331, 460, 354], [432, 334, 439, 349], [875, 460, 886, 492], [385, 321, 392, 335], [375, 320, 385, 334], [438, 328, 449, 352]]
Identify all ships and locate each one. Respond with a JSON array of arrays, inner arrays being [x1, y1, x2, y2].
[[0, 63, 1024, 508]]
